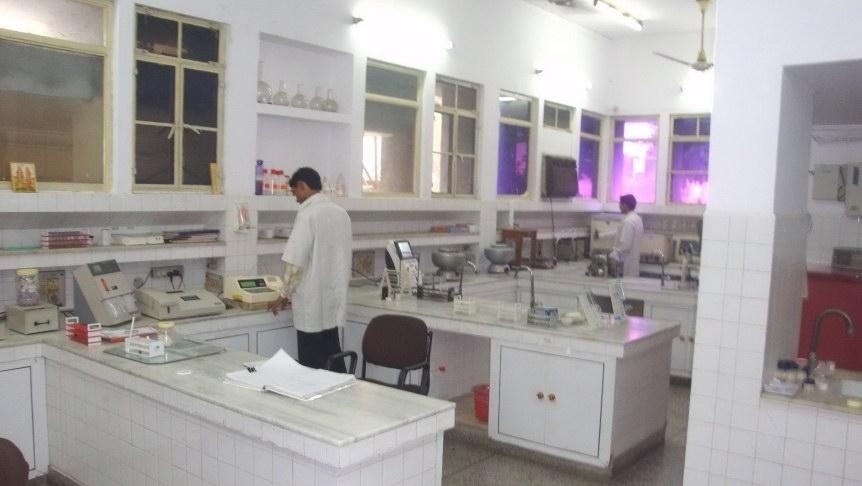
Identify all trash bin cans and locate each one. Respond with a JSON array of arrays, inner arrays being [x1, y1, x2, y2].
[[474, 382, 489, 422]]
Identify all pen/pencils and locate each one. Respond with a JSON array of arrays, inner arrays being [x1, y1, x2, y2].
[[129, 316, 135, 338]]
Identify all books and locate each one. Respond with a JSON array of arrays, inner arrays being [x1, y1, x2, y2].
[[221, 348, 357, 401], [41, 231, 90, 248], [161, 230, 221, 244]]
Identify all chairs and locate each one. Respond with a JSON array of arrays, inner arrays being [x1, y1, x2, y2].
[[324, 315, 432, 396]]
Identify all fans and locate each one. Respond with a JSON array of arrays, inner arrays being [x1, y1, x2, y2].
[[654, 0, 714, 71]]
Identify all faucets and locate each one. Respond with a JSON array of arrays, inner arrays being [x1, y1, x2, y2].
[[606, 248, 622, 268], [459, 260, 477, 293], [652, 248, 665, 272], [514, 265, 534, 298], [810, 310, 854, 352]]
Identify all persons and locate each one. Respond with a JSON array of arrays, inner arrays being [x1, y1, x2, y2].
[[268, 167, 354, 373], [609, 194, 643, 275]]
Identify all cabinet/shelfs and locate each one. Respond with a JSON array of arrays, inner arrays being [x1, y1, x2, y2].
[[486, 337, 675, 481], [202, 318, 408, 384], [646, 292, 698, 380]]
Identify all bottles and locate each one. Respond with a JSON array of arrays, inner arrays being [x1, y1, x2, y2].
[[157, 321, 177, 347], [14, 268, 41, 306], [256, 60, 339, 112], [254, 159, 346, 198]]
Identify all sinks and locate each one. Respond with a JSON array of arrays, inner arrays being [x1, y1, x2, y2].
[[842, 378, 862, 399]]
